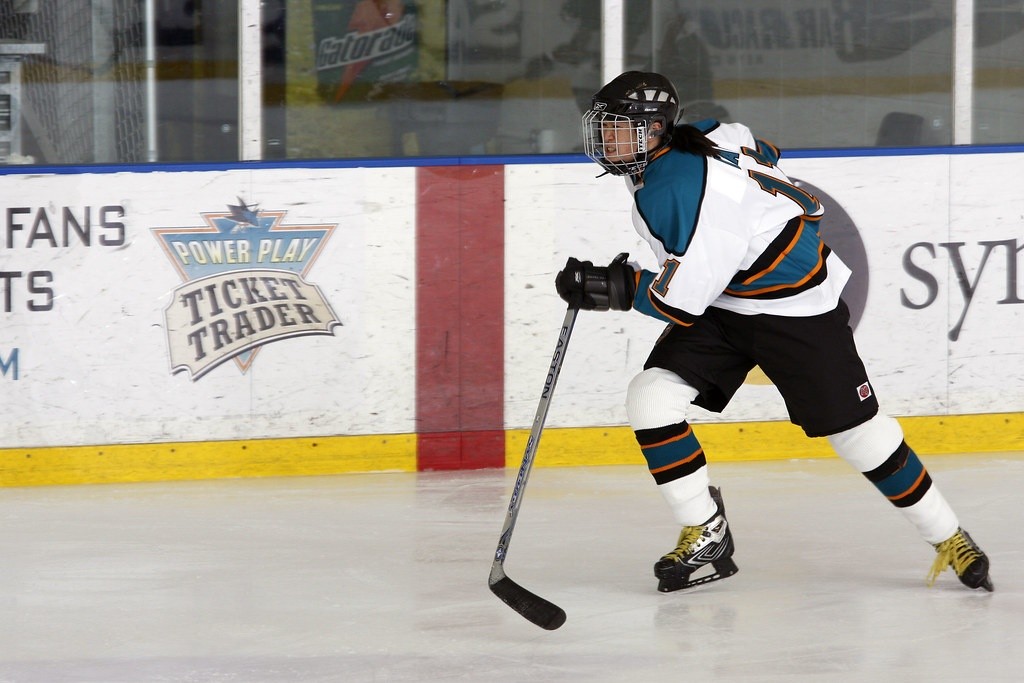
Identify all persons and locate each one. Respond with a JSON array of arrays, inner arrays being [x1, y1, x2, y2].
[[556, 71, 989, 589]]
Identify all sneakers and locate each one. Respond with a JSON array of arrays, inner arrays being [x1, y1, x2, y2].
[[654, 486, 738, 593], [925, 527, 994, 593]]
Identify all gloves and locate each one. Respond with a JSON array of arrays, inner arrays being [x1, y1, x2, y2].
[[555, 252, 635, 311]]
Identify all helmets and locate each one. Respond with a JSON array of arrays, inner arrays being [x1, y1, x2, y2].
[[581, 70, 680, 176]]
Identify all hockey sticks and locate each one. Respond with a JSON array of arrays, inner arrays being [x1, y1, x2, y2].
[[485, 257, 596, 633]]
